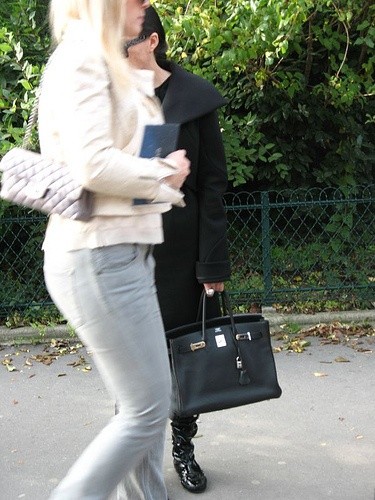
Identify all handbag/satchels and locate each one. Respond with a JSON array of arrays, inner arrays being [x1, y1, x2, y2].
[[167, 285, 283, 419], [0, 147, 94, 221]]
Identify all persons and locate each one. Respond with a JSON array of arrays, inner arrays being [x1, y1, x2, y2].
[[122, 4, 232, 491], [40, 2, 189, 500]]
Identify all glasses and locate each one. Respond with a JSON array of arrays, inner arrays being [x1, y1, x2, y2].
[[120, 35, 147, 58]]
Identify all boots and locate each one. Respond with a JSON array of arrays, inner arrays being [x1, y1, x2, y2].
[[167, 425, 208, 491]]
